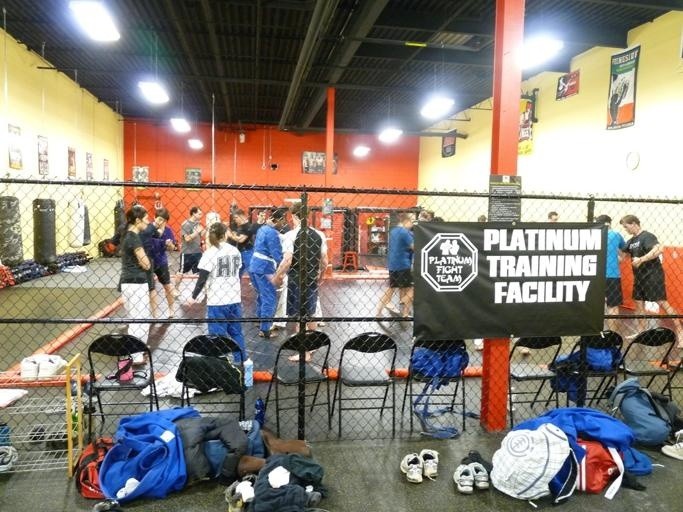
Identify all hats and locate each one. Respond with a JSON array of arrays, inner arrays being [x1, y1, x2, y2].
[[595, 215, 611, 225]]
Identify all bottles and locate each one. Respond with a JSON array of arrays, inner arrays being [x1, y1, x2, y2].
[[83, 405, 97, 434], [243, 355, 253, 387], [507, 376, 517, 411], [254, 396, 265, 429]]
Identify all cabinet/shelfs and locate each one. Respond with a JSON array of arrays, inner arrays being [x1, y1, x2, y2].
[[0, 352, 84, 478]]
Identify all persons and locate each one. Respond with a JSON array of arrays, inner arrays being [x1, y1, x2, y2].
[[548, 213, 560, 221], [376, 212, 444, 317], [478, 215, 487, 222], [596, 215, 682, 348], [474, 338, 531, 355], [119, 202, 328, 367]]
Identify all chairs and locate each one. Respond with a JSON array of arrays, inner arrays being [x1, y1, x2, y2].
[[507, 335, 563, 429], [547, 326, 683, 415], [88, 332, 159, 441], [175, 334, 246, 422], [330, 329, 399, 436], [401, 339, 468, 433], [260, 328, 332, 443]]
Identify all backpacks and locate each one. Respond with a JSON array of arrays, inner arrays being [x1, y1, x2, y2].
[[607, 376, 675, 448]]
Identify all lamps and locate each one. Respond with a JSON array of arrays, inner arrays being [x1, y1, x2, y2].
[[377, 94, 403, 144], [72, 2, 123, 46], [419, 49, 455, 121], [137, 28, 170, 104], [169, 80, 191, 134], [352, 104, 371, 160], [519, 1, 564, 69], [186, 109, 204, 150]]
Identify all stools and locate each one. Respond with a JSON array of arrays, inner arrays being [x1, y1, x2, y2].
[[342, 251, 357, 272]]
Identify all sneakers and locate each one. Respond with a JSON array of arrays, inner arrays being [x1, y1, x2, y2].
[[400, 448, 439, 483], [454, 450, 492, 494]]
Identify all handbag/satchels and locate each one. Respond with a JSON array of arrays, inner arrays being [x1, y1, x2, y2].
[[78, 437, 115, 499], [204, 419, 265, 480], [412, 347, 469, 378]]
